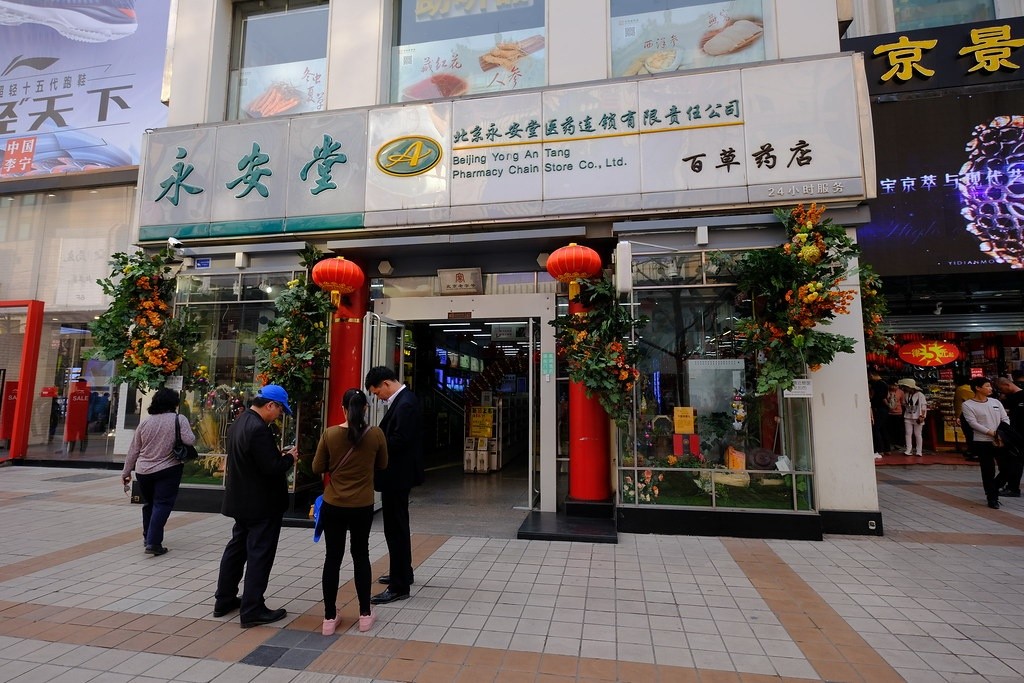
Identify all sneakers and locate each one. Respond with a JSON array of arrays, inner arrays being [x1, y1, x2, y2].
[[322, 608, 342, 636], [359, 611, 374, 631]]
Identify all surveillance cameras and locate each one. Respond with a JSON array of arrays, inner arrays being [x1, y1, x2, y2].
[[168, 237, 183, 249]]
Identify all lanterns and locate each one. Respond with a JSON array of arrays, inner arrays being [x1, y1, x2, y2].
[[311, 258, 364, 307], [546, 242, 601, 300]]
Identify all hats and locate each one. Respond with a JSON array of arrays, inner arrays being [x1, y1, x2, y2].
[[313, 495, 323, 543], [256, 384, 292, 415], [895, 378, 924, 393]]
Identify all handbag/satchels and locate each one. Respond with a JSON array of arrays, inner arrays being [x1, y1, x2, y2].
[[172, 414, 198, 461]]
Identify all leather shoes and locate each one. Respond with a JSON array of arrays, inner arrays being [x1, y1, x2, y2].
[[371, 588, 409, 604], [379, 576, 414, 585]]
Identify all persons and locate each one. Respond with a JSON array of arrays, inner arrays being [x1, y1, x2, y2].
[[214, 385, 298, 628], [49, 378, 110, 457], [121, 388, 195, 554], [867, 366, 1024, 497], [365, 366, 426, 603], [962, 377, 1010, 509], [313, 388, 388, 635]]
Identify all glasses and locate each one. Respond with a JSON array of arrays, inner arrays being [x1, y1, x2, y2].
[[124, 479, 134, 498]]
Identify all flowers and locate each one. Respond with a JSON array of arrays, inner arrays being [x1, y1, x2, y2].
[[547, 275, 649, 436], [619, 452, 729, 504], [705, 201, 897, 394], [79, 243, 338, 484]]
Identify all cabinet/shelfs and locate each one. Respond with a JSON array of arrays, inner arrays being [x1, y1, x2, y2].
[[463, 394, 526, 472]]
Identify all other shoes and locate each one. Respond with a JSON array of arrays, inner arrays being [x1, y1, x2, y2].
[[987, 500, 1002, 509], [998, 488, 1020, 497], [904, 450, 913, 455], [241, 604, 287, 628], [874, 452, 882, 459], [916, 450, 922, 457], [213, 595, 243, 617], [145, 546, 167, 555]]
